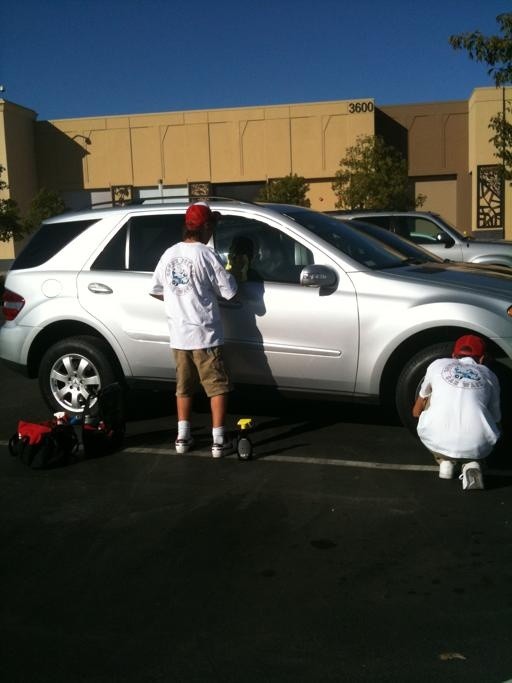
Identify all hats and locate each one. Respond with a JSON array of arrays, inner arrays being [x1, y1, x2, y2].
[[453, 334, 485, 358], [184, 199, 222, 233]]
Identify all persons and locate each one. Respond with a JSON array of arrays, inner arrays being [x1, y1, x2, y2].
[[147, 200, 242, 458], [411, 329, 505, 491]]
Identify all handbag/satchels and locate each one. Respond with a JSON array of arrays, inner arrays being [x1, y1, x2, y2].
[[7, 412, 128, 481]]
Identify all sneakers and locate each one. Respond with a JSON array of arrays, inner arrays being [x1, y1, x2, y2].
[[437, 458, 456, 480], [460, 461, 484, 491], [210, 442, 224, 458], [174, 435, 195, 453]]
